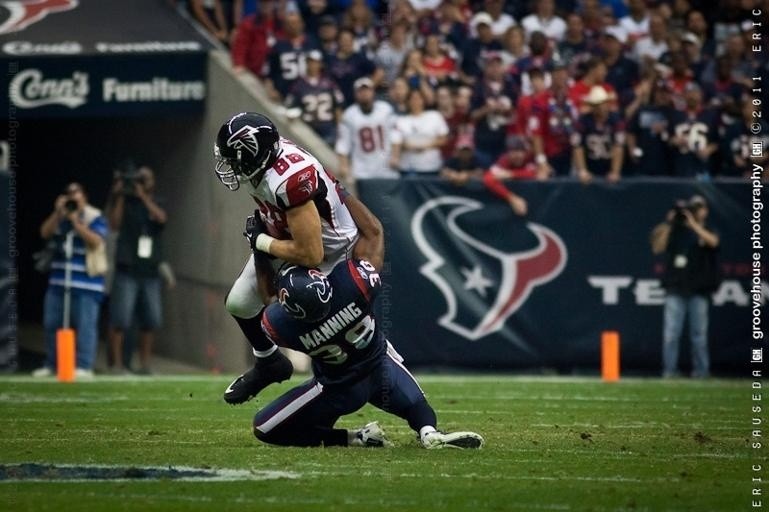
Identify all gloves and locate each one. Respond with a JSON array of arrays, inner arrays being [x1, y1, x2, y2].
[[324, 167, 351, 204], [243, 209, 269, 259]]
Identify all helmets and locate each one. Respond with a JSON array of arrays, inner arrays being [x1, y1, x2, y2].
[[274, 261, 334, 324], [212, 112, 279, 191]]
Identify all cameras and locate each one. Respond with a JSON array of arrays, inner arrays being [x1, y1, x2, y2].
[[66, 199, 78, 210]]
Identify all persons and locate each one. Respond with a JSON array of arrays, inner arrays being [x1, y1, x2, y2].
[[650, 193, 723, 379], [184, 0, 768, 217], [27, 182, 112, 378], [208, 112, 403, 405], [101, 164, 167, 380], [155, 242, 177, 291], [253, 184, 487, 455]]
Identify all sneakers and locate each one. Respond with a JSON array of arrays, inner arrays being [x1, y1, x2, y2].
[[420, 429, 484, 451], [351, 422, 390, 447], [224, 357, 293, 406]]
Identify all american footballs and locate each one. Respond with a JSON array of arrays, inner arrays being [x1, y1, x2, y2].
[[261, 219, 290, 260]]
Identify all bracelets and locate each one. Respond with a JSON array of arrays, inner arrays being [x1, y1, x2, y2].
[[255, 232, 275, 254]]
